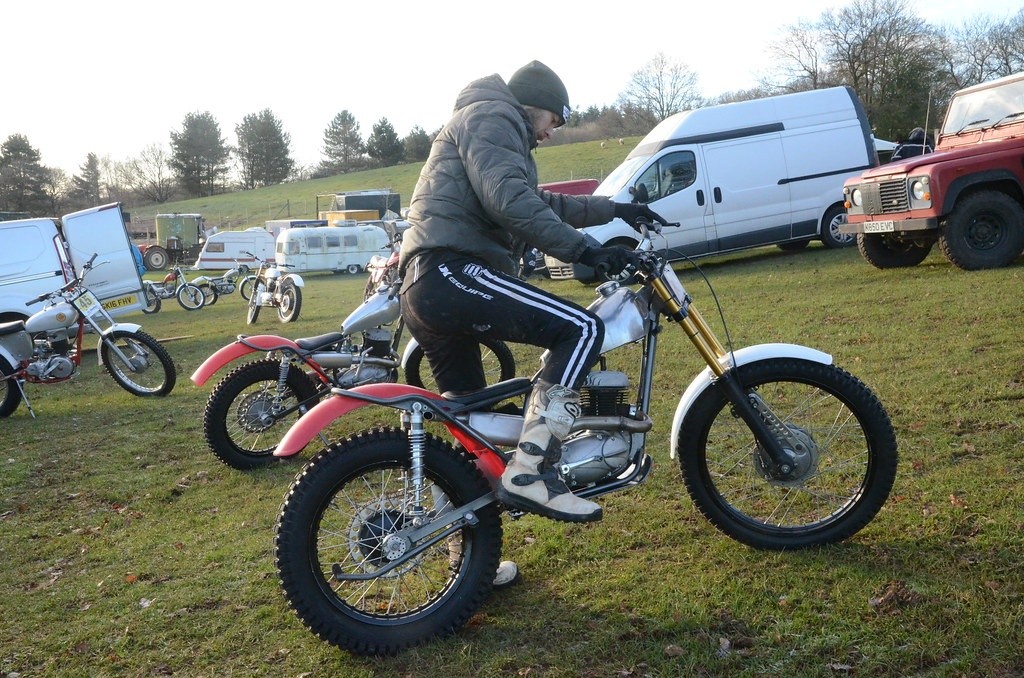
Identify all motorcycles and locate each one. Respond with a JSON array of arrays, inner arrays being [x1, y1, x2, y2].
[[190, 258, 265, 306], [244, 249, 306, 325], [0, 253, 179, 417], [269, 183, 899, 654], [141, 258, 205, 315], [193, 231, 519, 470]]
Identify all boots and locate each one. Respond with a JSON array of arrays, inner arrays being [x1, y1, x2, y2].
[[426, 482, 520, 593], [498, 381, 602, 524]]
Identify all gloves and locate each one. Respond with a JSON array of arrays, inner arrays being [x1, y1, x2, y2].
[[612, 201, 666, 237], [583, 247, 635, 276]]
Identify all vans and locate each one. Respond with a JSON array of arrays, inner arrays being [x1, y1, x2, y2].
[[0, 200, 153, 358], [543, 84, 880, 288], [135, 177, 600, 276]]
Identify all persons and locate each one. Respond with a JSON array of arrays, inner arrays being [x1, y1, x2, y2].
[[398, 58, 668, 579], [891, 126, 934, 162]]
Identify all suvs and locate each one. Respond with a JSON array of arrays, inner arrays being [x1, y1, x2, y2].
[[836, 69, 1023, 271]]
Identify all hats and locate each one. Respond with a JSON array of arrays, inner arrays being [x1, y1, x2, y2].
[[507, 62, 572, 131]]
